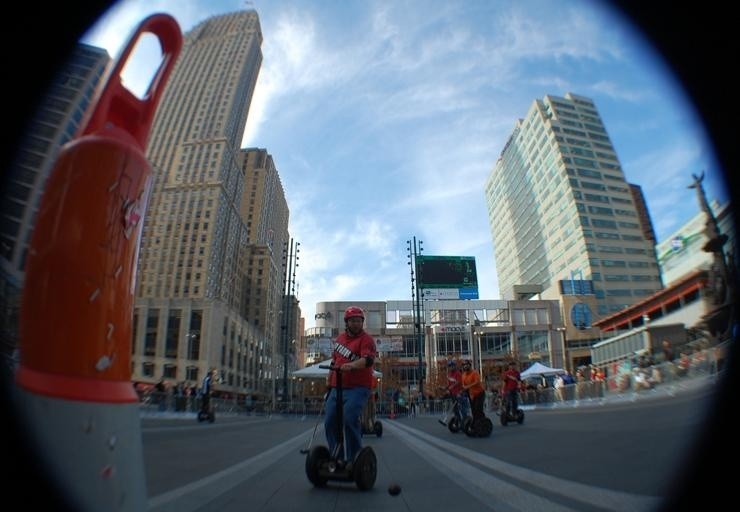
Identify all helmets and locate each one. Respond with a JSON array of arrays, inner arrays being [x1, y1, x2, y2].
[[447, 359, 456, 366], [343, 305, 365, 321]]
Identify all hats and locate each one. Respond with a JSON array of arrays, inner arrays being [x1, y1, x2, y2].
[[462, 360, 472, 366]]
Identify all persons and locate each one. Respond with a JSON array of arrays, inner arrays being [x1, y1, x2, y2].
[[201, 372, 213, 413], [324, 307, 376, 464], [157, 376, 201, 411], [502, 360, 523, 417], [519, 329, 728, 403], [462, 359, 484, 427], [416, 392, 426, 414], [438, 361, 468, 420]]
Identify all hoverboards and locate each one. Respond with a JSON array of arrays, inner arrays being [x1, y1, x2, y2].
[[302, 363, 383, 491], [437, 388, 528, 440], [196, 409, 218, 424]]
